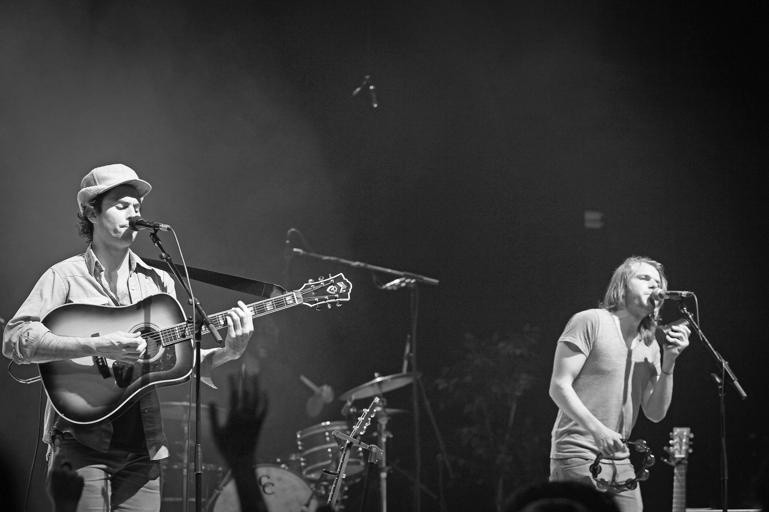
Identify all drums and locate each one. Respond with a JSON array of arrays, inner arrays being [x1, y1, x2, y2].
[[297, 420, 366, 479], [213, 464, 318, 511]]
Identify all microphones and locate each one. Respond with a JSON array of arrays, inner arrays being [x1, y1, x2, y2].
[[130, 216, 170, 233], [650, 288, 693, 302]]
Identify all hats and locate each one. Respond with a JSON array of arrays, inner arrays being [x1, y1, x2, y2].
[[76, 164, 153, 217]]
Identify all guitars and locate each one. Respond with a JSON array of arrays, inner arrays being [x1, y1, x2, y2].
[[37, 271, 353, 431]]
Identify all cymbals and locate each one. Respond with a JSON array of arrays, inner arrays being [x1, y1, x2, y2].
[[360, 408, 405, 415], [338, 371, 422, 402], [159, 402, 225, 422]]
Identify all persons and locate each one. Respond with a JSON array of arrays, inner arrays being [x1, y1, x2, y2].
[[548, 255, 691, 512], [1, 163, 254, 512]]
